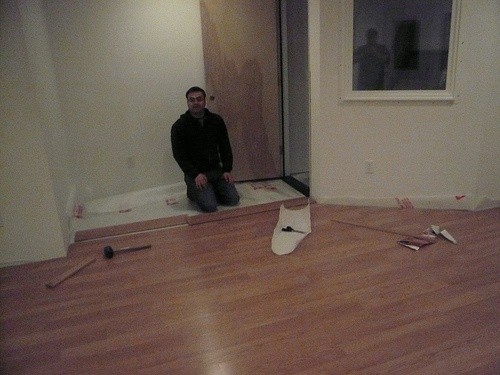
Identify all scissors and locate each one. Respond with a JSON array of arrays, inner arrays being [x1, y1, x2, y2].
[[282, 224, 305, 234]]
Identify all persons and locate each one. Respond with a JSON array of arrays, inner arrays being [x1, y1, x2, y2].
[[170, 86, 240, 213], [353, 26, 391, 90]]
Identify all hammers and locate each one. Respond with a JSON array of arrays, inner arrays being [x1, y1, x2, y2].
[[104, 242, 151, 258]]
